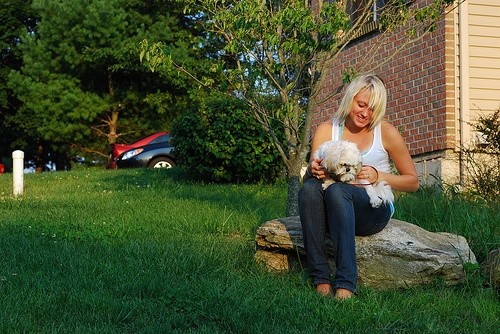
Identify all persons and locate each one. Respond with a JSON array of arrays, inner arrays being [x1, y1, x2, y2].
[[297, 75, 420, 300]]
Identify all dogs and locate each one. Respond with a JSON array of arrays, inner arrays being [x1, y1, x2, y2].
[[314, 139, 393, 208]]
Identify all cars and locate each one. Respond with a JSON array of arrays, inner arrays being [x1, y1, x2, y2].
[[106, 130, 180, 170]]
